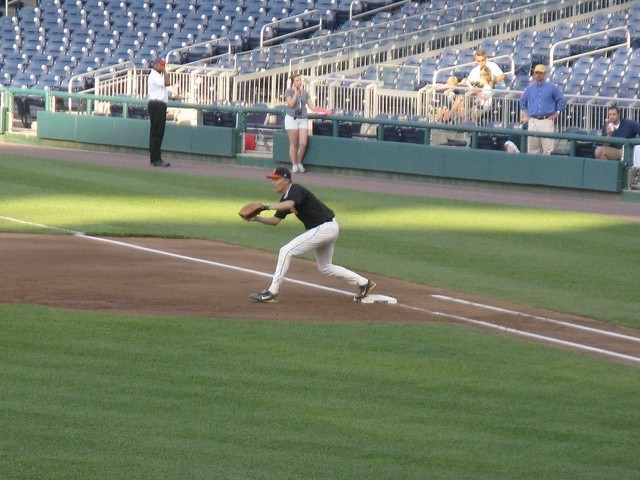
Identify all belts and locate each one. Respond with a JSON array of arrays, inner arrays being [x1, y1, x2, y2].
[[531, 115, 548, 120]]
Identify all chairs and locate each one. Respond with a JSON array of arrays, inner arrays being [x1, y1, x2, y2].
[[0, 2, 640, 154]]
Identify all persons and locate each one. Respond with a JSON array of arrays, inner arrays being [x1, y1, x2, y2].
[[247, 166, 377, 304], [474, 65, 492, 112], [467, 51, 506, 89], [147, 59, 172, 168], [595, 106, 640, 161], [519, 64, 567, 156], [442, 76, 459, 108], [284, 74, 334, 173]]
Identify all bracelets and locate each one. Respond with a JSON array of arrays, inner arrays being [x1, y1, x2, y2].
[[266, 203, 270, 210]]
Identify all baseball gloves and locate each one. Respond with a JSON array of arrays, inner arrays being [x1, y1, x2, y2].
[[239, 203, 270, 220]]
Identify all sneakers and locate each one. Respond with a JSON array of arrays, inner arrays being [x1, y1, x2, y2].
[[352, 279, 376, 301], [249, 291, 278, 303], [150, 159, 170, 167], [298, 164, 306, 172], [292, 165, 300, 172]]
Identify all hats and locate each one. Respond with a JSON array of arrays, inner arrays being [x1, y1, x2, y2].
[[447, 76, 458, 84], [266, 167, 291, 179], [535, 64, 547, 72]]
